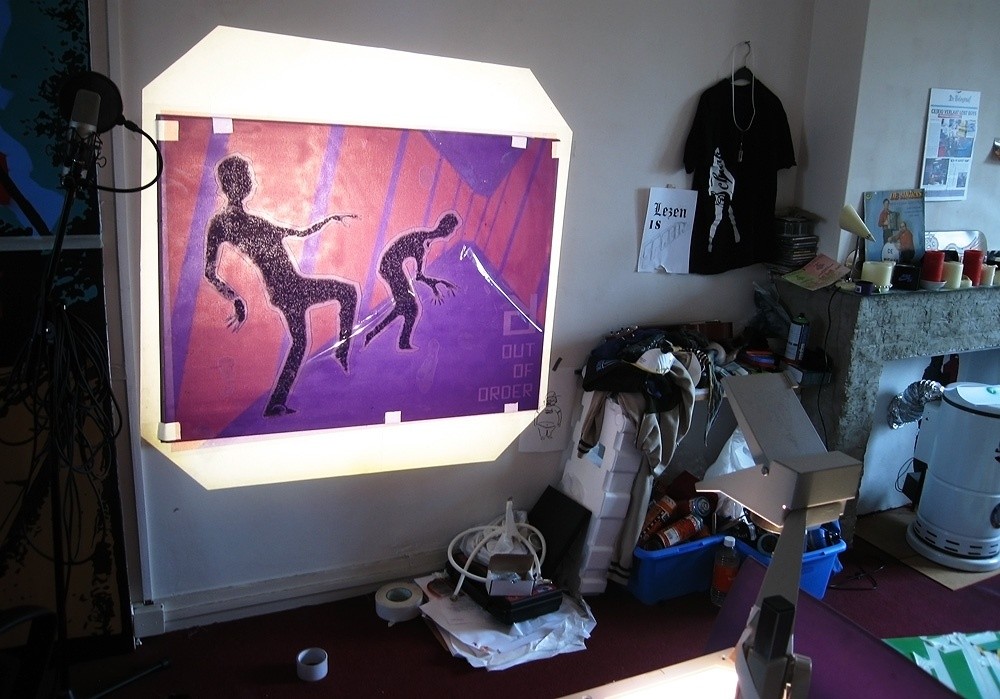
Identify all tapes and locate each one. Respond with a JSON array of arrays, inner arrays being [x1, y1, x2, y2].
[[375, 581, 424, 623], [296, 647, 329, 680]]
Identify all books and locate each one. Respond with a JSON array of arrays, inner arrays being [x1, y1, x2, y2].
[[862, 188, 925, 277]]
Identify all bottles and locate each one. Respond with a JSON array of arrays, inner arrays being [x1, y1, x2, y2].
[[710, 536, 741, 608], [656, 514, 704, 549], [673, 498, 711, 517], [806, 527, 838, 553], [784, 313, 809, 365]]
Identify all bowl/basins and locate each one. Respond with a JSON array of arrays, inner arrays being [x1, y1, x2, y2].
[[920, 280, 947, 291]]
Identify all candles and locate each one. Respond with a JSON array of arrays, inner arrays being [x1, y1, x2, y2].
[[861, 261, 891, 287], [922, 251, 945, 281], [963, 250, 985, 286], [941, 261, 964, 289], [980, 263, 998, 286]]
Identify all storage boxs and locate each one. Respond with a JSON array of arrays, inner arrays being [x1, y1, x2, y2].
[[632, 533, 723, 604], [736, 536, 847, 602], [446, 553, 563, 625]]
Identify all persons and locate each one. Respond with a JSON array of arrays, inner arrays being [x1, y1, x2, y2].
[[888, 221, 915, 264], [877, 198, 895, 246]]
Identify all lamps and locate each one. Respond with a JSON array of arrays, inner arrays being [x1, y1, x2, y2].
[[834, 205, 877, 291], [696, 371, 862, 699]]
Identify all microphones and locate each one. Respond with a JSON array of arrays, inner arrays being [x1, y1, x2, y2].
[[66, 89, 102, 160]]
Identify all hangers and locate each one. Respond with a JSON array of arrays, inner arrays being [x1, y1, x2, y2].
[[728, 41, 756, 83]]
